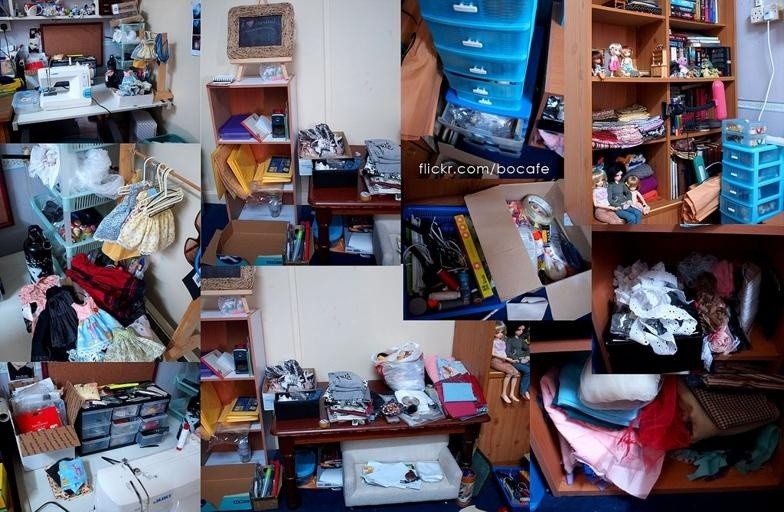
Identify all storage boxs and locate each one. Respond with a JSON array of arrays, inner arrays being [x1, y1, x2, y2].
[[721, 211, 742, 224], [428, 141, 499, 180], [721, 177, 784, 206], [6, 380, 85, 471], [722, 144, 784, 168], [144, 134, 186, 144], [721, 161, 784, 187], [438, 25, 545, 153], [170, 398, 190, 420], [722, 118, 768, 147], [110, 87, 154, 107], [201, 220, 315, 266], [276, 390, 322, 421], [422, 15, 536, 61], [457, 90, 522, 112], [76, 382, 171, 456], [443, 69, 524, 100], [419, 0, 539, 29], [401, 206, 552, 320], [176, 375, 199, 397], [201, 465, 283, 509], [462, 140, 528, 159], [464, 180, 592, 320], [313, 161, 357, 189], [493, 465, 530, 511], [433, 42, 528, 83], [720, 194, 783, 223], [12, 90, 40, 114]]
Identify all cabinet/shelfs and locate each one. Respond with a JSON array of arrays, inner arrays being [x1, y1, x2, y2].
[[270, 378, 491, 512], [453, 321, 530, 466], [310, 143, 403, 249], [528, 339, 784, 496], [29, 144, 120, 287], [201, 309, 276, 466], [206, 74, 300, 228], [0, 0, 139, 20], [592, 229, 784, 379], [116, 22, 146, 71], [563, 1, 738, 226]]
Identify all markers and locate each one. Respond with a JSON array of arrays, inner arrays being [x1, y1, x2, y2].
[[286, 221, 309, 261], [254, 464, 277, 499]]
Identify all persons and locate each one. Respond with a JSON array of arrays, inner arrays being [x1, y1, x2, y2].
[[28, 37, 40, 53], [490, 319, 521, 404], [8, 361, 34, 381]]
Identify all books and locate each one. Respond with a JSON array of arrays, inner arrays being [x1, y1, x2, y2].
[[669, 0, 719, 24], [669, 33, 721, 57]]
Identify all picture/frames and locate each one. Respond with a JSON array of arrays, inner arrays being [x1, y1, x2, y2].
[[227, 2, 295, 59], [202, 267, 255, 290]]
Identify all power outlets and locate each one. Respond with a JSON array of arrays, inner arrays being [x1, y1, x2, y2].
[[765, 3, 780, 21], [0, 20, 12, 32], [751, 7, 765, 24]]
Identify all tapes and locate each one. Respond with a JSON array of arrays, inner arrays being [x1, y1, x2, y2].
[[358, 189, 375, 204], [521, 194, 556, 225], [319, 418, 330, 429]]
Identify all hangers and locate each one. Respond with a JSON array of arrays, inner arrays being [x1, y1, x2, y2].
[[118, 157, 184, 217]]
